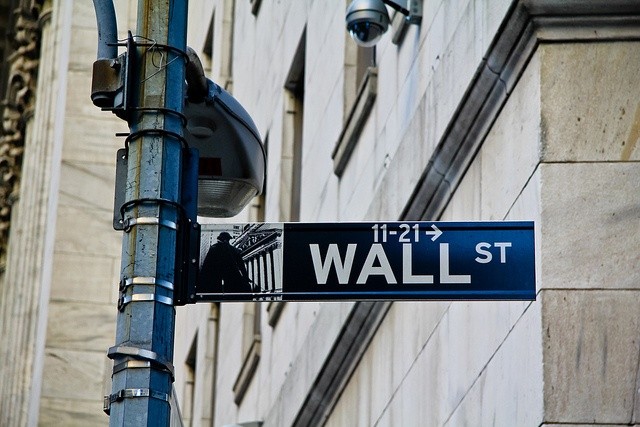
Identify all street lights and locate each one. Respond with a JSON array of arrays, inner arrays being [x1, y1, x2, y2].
[[103, 45, 266, 427]]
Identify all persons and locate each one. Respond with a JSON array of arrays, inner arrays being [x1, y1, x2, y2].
[[199, 232, 253, 291]]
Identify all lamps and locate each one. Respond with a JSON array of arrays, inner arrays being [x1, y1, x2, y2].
[[344, 0, 423, 49]]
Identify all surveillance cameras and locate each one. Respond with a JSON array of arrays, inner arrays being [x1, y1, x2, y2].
[[343, 0, 390, 49]]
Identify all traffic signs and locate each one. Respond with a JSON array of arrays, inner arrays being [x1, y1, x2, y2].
[[195, 222, 536, 301]]
